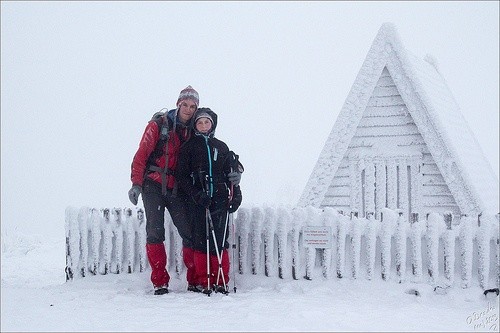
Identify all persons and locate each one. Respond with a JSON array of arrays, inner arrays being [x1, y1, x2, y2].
[[175, 108, 242, 294], [129, 86, 241, 295]]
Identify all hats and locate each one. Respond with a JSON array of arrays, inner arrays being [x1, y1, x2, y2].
[[175, 86, 199, 118], [195, 113, 214, 124]]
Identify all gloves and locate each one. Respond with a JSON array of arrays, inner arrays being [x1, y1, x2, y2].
[[227, 185, 242, 213], [128, 184, 140, 206], [228, 172, 241, 188], [194, 191, 208, 208]]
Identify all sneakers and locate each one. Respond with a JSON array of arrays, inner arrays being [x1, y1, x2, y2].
[[187, 283, 212, 294], [154, 287, 168, 295]]
